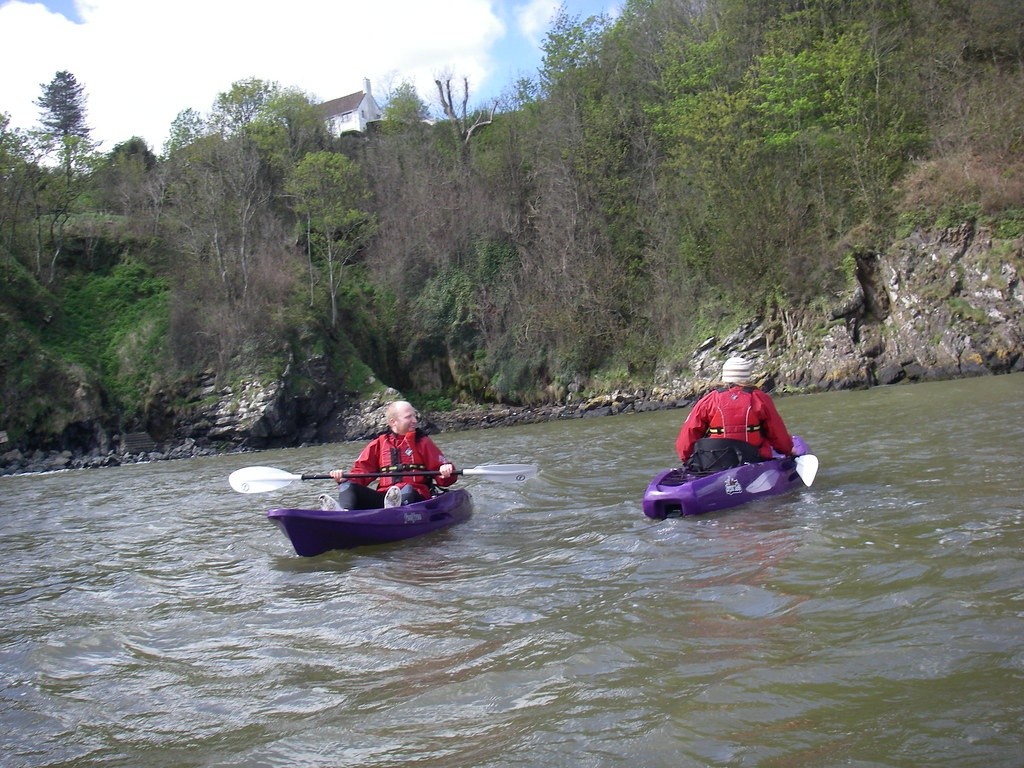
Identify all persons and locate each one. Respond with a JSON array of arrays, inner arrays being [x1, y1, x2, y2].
[[317, 401, 458, 511], [675, 357, 795, 462]]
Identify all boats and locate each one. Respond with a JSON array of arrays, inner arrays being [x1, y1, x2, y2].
[[643, 436, 809, 520], [267, 485, 473, 558]]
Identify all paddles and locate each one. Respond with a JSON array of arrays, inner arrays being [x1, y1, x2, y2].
[[227, 462, 539, 495], [777, 449, 820, 488]]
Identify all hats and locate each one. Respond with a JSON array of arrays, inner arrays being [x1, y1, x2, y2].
[[722, 356, 752, 383]]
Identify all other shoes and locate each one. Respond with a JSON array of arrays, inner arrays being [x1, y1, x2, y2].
[[384, 485, 401, 508], [318, 494, 344, 511]]
